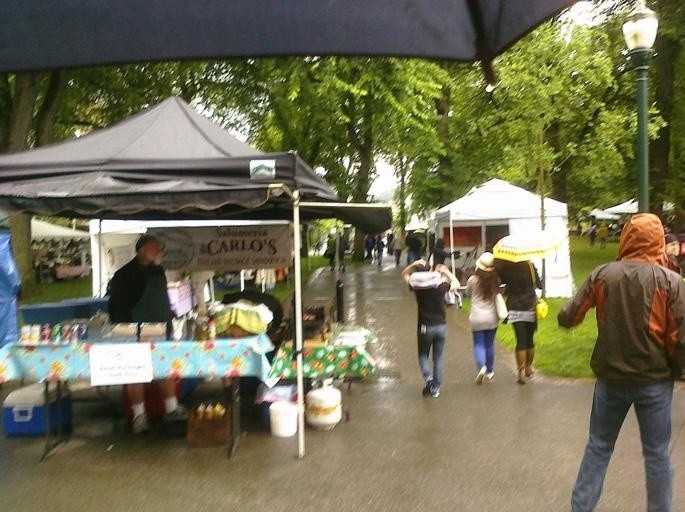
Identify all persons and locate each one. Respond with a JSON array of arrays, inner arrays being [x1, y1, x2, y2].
[[464, 251, 508, 384], [402, 257, 460, 400], [324, 228, 461, 273], [557, 211, 684, 511], [496, 260, 544, 386], [106, 235, 186, 437], [567, 211, 684, 281]]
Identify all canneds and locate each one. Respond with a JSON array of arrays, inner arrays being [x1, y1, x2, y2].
[[20, 323, 88, 345]]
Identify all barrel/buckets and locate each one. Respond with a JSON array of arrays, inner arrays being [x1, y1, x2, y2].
[[270, 401, 298, 438]]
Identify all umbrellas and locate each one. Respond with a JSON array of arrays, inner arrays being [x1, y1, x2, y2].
[[490, 231, 563, 264]]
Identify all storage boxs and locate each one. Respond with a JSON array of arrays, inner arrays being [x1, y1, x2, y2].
[[3, 382, 71, 436]]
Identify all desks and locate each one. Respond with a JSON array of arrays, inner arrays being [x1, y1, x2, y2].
[[277, 323, 377, 424], [0, 332, 279, 461]]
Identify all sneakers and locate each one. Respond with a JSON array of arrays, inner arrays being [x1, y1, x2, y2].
[[163, 403, 188, 422], [488, 371, 495, 379], [475, 365, 487, 385], [422, 376, 437, 397], [432, 388, 441, 398], [130, 411, 149, 435]]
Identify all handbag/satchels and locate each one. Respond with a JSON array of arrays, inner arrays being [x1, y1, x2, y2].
[[536, 298, 549, 320], [495, 293, 509, 321]]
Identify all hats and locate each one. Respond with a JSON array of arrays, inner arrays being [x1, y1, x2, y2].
[[476, 251, 496, 272]]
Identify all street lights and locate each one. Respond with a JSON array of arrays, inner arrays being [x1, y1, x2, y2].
[[622, 0, 660, 221]]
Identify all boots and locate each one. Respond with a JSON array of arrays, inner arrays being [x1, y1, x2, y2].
[[526, 348, 535, 376], [516, 350, 527, 384]]
